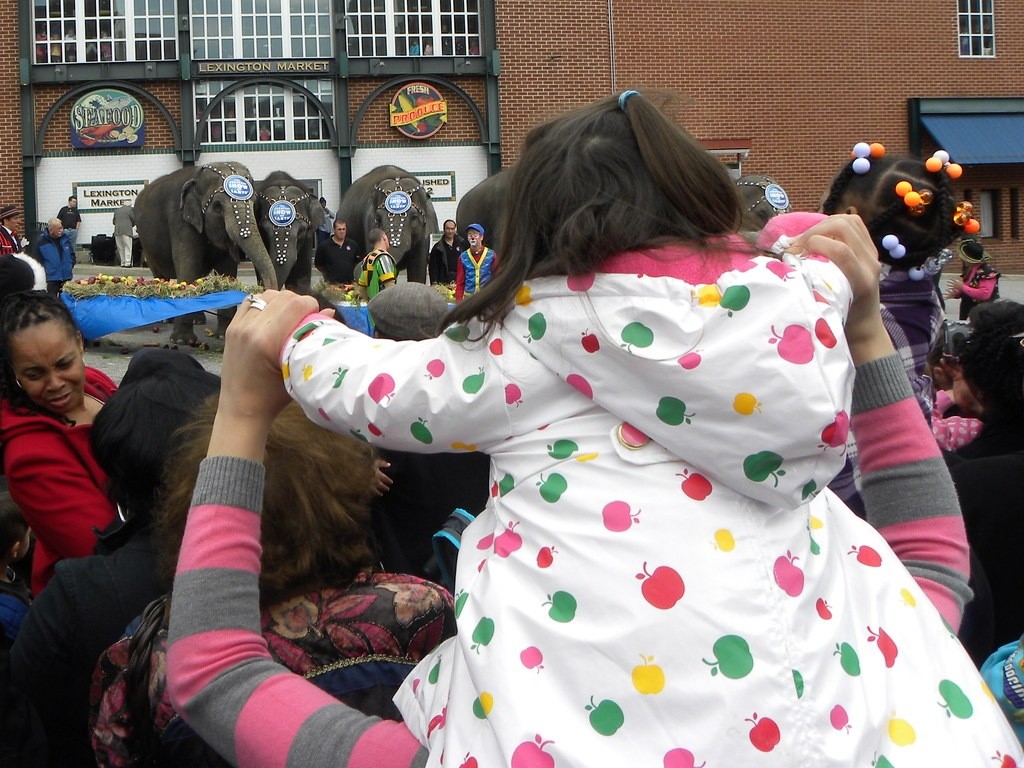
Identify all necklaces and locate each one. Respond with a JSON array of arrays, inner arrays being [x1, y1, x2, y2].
[[85, 393, 106, 406]]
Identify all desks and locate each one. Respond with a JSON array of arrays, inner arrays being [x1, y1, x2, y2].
[[56, 289, 457, 342]]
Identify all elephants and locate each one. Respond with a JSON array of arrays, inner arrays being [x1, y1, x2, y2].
[[136, 161, 324, 296], [736, 174, 793, 232], [337, 165, 438, 285], [456, 165, 510, 262]]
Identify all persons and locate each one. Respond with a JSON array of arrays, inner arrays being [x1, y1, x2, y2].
[[113, 200, 137, 268], [0, 93, 1024, 768]]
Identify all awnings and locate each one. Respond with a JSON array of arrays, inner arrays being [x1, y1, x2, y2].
[[921, 114, 1024, 163]]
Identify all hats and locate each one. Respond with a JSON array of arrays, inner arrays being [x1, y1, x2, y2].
[[368, 282, 447, 339], [465, 224, 484, 234], [0, 205, 19, 219]]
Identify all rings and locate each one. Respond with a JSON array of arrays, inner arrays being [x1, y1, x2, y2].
[[249, 303, 263, 311], [244, 294, 257, 304], [251, 297, 267, 307]]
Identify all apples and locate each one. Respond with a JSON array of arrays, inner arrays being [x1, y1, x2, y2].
[[339, 284, 354, 301], [447, 289, 456, 300], [76, 275, 223, 352]]
